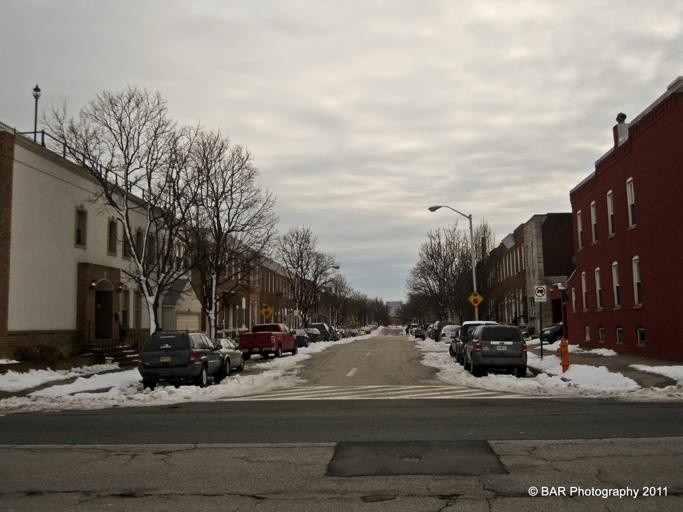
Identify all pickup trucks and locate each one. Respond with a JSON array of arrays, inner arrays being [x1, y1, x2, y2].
[[238, 323, 298, 359]]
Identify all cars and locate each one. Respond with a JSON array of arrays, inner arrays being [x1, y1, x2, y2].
[[539, 321, 564, 344], [289, 322, 373, 345], [405, 322, 528, 377], [212, 338, 244, 375]]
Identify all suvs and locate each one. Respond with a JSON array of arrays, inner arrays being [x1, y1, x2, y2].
[[137, 329, 224, 388]]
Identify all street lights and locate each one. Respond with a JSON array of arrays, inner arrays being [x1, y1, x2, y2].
[[31, 85, 41, 142], [428, 205, 480, 322]]
